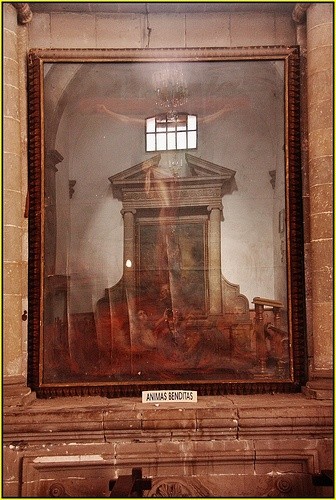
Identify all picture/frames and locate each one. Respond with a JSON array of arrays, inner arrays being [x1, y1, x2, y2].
[[25, 46, 308, 397]]
